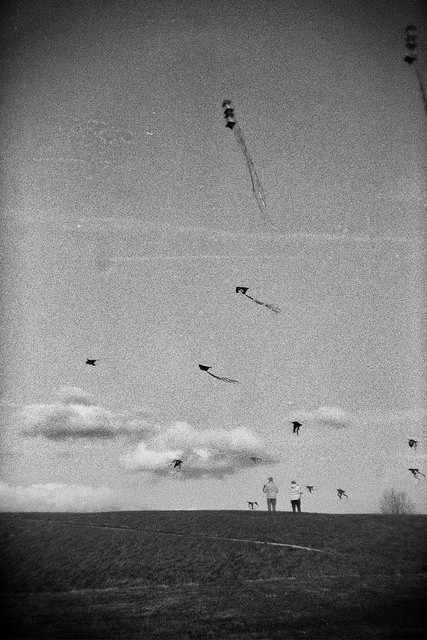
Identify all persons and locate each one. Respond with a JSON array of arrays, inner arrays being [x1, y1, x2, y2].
[[289, 481, 301, 511], [263, 477, 278, 512]]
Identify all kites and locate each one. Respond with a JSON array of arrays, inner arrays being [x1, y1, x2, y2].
[[307, 486, 313, 493], [403, 24, 427, 118], [86, 359, 96, 366], [222, 100, 266, 214], [409, 440, 416, 447], [408, 468, 425, 479], [292, 421, 302, 435], [337, 489, 348, 499], [199, 364, 238, 382], [236, 287, 280, 313], [174, 460, 183, 468], [248, 502, 257, 509]]
